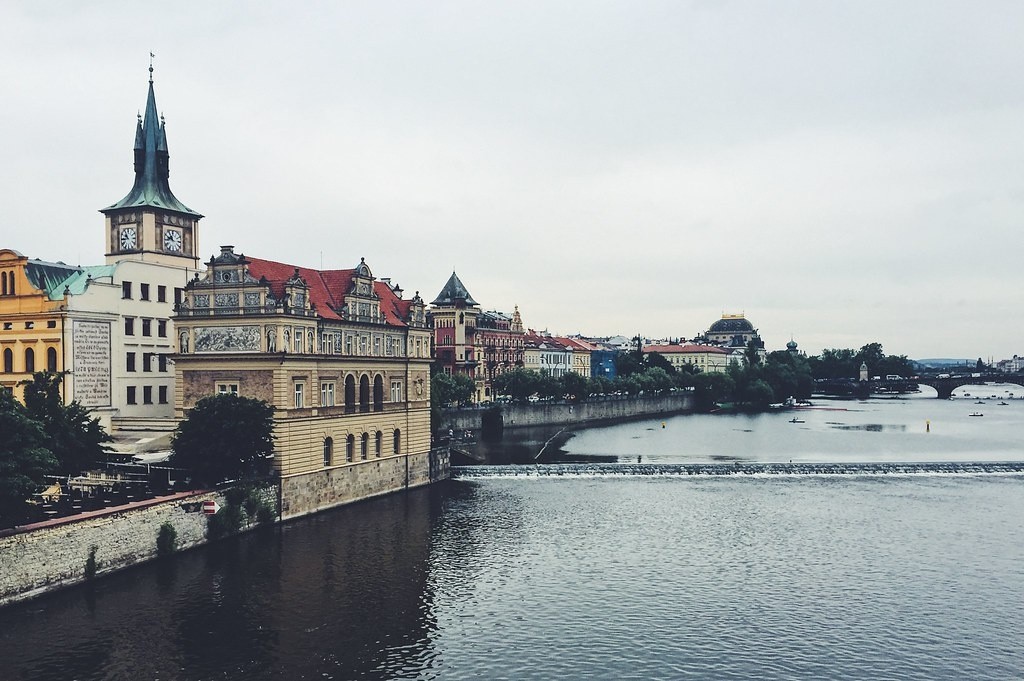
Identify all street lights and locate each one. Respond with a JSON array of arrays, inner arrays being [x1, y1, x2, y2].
[[576, 358, 585, 377], [708, 360, 716, 373], [541, 357, 551, 376], [553, 361, 562, 377]]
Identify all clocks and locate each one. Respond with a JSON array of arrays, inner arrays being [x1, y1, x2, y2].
[[121, 228, 136, 249], [164, 229, 181, 251]]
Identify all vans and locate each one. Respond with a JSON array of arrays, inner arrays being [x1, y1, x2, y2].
[[886, 375, 903, 382], [870, 376, 880, 381]]
